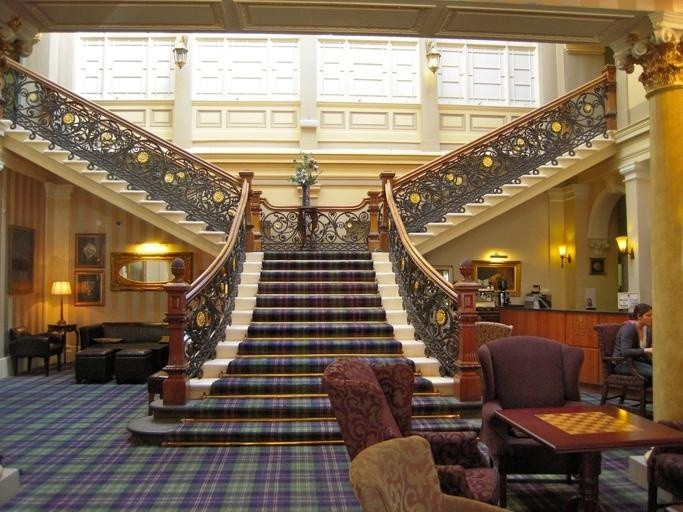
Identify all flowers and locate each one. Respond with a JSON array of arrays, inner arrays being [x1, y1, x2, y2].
[[287, 150, 324, 187]]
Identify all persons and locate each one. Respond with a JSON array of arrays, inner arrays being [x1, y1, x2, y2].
[[613, 304, 652, 378]]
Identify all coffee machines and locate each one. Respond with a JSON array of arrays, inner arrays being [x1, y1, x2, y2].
[[498, 280, 510, 307]]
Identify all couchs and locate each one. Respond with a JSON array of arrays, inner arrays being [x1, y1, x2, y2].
[[79, 321, 170, 374]]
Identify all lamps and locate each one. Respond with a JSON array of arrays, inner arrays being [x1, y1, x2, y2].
[[173, 32, 189, 71], [490, 252, 507, 258], [427, 39, 441, 77], [50, 280, 72, 325], [559, 245, 571, 268], [615, 236, 634, 258]]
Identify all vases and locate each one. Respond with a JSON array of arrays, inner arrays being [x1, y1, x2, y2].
[[302, 186, 311, 209]]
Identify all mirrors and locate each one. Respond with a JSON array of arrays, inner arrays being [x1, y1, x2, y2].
[[110, 251, 194, 291]]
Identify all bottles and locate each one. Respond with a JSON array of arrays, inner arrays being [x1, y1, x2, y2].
[[476, 292, 491, 301], [487, 285, 494, 290]]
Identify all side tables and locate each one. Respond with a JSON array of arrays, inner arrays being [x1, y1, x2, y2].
[[49, 324, 78, 363]]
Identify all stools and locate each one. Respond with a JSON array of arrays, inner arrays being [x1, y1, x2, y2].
[[75, 347, 113, 383], [114, 348, 152, 384]]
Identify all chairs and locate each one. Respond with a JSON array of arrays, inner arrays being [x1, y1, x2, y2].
[[9, 326, 65, 377]]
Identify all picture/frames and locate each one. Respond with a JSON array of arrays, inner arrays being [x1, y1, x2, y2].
[[75, 269, 106, 307], [473, 261, 522, 296], [74, 231, 105, 268], [432, 265, 453, 285], [9, 225, 37, 295]]
